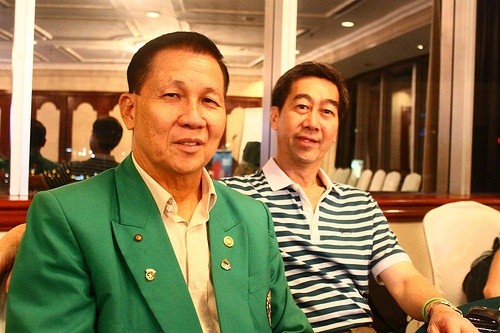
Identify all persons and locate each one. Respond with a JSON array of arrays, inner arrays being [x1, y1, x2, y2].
[[28, 116, 123, 191], [0, 119, 59, 177], [5, 31, 311, 332], [215, 62, 479, 332]]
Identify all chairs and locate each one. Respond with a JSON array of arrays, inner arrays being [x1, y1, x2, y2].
[[329, 168, 421, 192], [423, 201, 500, 308]]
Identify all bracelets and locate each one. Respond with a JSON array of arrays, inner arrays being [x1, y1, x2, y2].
[[423, 297, 464, 324]]
